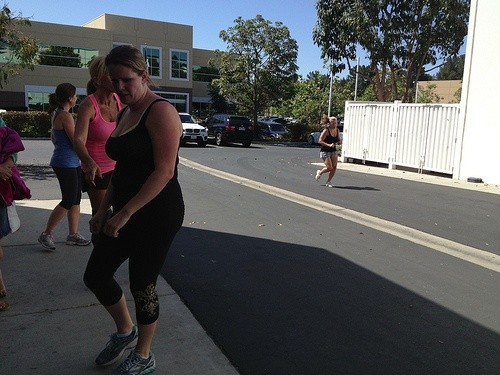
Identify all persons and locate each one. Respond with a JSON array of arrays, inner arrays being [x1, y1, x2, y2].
[[73, 56, 128, 247], [38, 83, 91, 251], [315, 117, 343, 187], [84, 45, 185, 375], [0, 114, 20, 311]]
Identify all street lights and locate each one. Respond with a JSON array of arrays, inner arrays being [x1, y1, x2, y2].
[[351, 56, 360, 100]]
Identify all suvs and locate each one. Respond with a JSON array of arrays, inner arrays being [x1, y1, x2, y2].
[[178, 112, 209, 147], [201, 114, 255, 148]]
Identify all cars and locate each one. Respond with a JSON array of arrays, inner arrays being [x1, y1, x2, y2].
[[249, 114, 301, 142], [305, 124, 345, 145]]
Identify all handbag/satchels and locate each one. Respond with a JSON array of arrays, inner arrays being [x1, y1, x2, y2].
[[7, 201, 20, 233]]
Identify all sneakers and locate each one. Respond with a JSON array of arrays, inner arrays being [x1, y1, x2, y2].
[[113, 350, 156, 375], [66, 232, 91, 246], [95, 324, 138, 366], [326, 181, 333, 188], [316, 169, 321, 181], [38, 232, 56, 250]]
